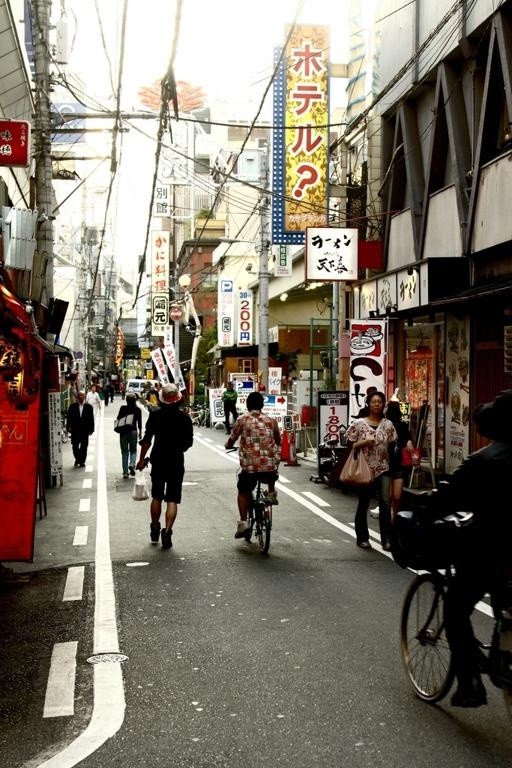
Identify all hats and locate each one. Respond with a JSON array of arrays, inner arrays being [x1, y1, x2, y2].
[[158, 384, 184, 404]]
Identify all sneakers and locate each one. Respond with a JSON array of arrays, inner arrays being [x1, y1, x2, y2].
[[360, 542, 371, 548], [123, 465, 135, 479], [234, 520, 252, 538], [266, 491, 280, 507], [382, 542, 391, 552]]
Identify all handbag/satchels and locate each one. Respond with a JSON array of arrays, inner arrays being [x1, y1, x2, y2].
[[401, 447, 421, 466]]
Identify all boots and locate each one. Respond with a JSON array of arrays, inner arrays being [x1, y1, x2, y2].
[[161, 528, 172, 549], [150, 521, 160, 543]]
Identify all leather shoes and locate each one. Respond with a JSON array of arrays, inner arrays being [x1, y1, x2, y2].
[[450, 678, 488, 708]]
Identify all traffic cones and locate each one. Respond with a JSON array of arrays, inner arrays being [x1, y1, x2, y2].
[[284, 432, 301, 466], [280, 431, 290, 461]]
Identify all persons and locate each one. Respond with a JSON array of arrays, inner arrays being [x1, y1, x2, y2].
[[342, 391, 398, 553], [401, 387, 512, 709], [85, 381, 161, 416], [114, 392, 143, 479], [223, 390, 283, 539], [134, 382, 195, 548], [221, 380, 238, 435], [66, 390, 95, 468], [385, 399, 415, 519]]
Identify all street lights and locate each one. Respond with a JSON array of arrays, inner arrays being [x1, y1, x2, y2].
[[179, 272, 202, 407]]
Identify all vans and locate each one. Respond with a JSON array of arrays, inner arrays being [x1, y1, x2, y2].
[[124, 378, 161, 400]]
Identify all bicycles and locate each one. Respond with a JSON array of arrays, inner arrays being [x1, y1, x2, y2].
[[318, 432, 344, 493], [183, 402, 210, 427], [226, 445, 280, 555], [388, 482, 511, 716]]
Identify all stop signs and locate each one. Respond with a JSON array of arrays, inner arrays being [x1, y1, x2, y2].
[[168, 305, 183, 323]]
